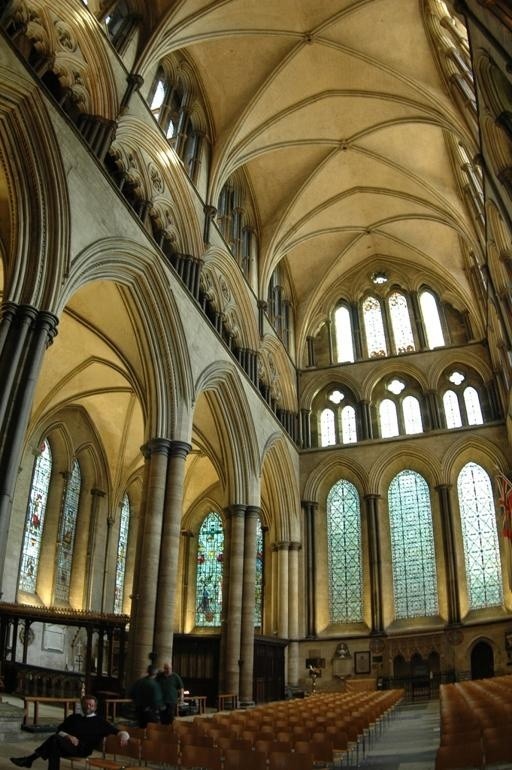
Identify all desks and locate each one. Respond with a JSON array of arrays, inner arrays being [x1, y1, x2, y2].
[[20, 695, 81, 727]]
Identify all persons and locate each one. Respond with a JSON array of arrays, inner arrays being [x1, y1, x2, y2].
[[10, 695, 129, 769], [159, 664, 185, 725], [133, 665, 166, 726]]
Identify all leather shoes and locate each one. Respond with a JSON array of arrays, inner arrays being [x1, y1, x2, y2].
[[10, 756, 33, 768]]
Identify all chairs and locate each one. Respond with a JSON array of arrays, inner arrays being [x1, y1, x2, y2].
[[60, 675, 406, 769], [435, 674, 510, 769]]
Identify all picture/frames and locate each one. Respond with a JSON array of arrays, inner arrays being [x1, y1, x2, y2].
[[354, 650, 371, 675]]
